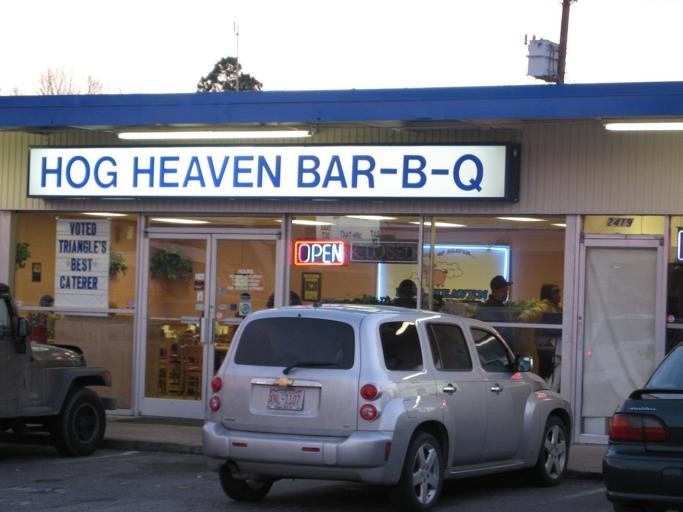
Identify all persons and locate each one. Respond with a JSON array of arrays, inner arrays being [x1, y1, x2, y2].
[[38, 295, 52, 306], [477, 276, 514, 366], [394, 280, 416, 307], [515, 284, 562, 379]]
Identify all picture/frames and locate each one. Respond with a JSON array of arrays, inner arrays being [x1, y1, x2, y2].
[[302, 272, 322, 301]]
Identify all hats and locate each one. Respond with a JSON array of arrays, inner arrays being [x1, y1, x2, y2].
[[490, 276, 513, 290]]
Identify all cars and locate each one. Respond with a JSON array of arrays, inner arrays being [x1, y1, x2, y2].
[[595, 341, 681, 512]]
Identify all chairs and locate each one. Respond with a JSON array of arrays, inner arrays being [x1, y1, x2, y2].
[[160, 335, 202, 400]]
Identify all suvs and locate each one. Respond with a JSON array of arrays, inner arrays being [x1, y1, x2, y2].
[[0, 279, 119, 463]]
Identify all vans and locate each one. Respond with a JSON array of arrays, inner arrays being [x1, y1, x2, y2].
[[194, 299, 587, 511]]
